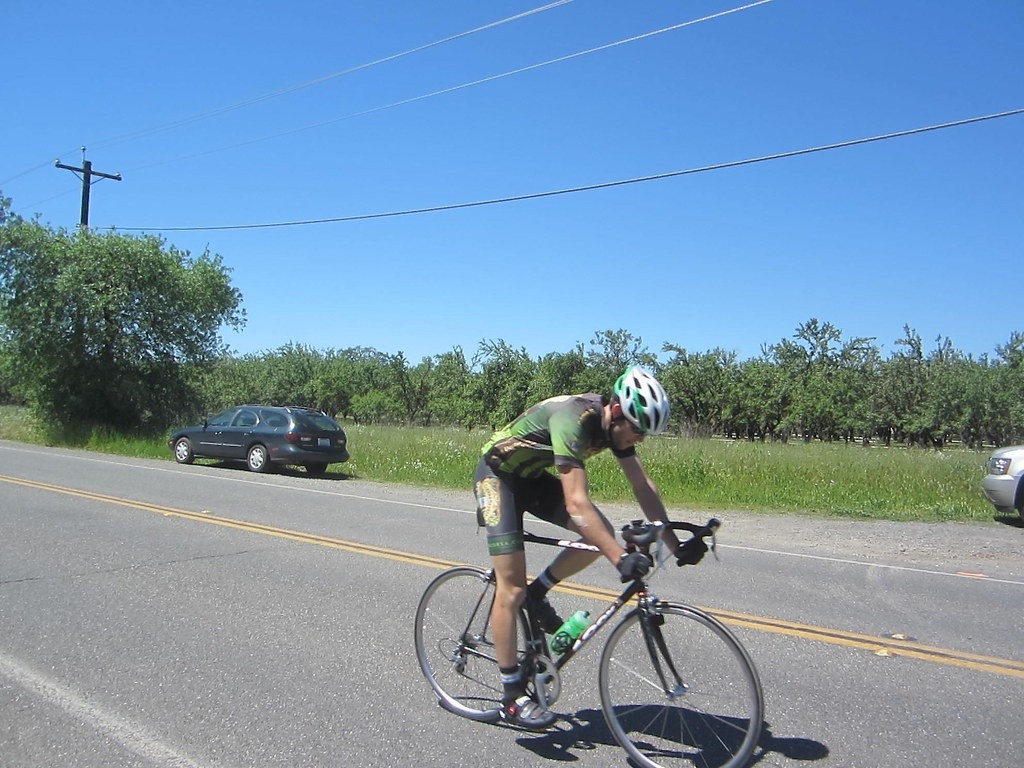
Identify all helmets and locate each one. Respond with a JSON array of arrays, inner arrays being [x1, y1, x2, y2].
[[614, 364, 670, 435]]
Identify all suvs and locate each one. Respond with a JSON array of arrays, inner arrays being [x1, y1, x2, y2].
[[980, 443, 1024, 526], [169, 401, 349, 476]]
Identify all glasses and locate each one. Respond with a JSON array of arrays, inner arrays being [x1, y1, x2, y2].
[[623, 416, 648, 437]]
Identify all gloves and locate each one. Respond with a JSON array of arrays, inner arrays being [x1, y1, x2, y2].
[[677, 536, 708, 567], [616, 553, 652, 581]]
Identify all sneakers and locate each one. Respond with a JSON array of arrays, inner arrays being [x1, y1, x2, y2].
[[521, 585, 564, 635], [500, 694, 556, 729]]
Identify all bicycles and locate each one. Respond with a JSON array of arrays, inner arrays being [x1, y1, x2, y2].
[[414, 508, 765, 768]]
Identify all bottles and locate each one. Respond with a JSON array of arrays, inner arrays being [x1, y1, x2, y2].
[[546, 610, 592, 658]]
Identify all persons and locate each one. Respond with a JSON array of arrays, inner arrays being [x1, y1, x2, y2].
[[473, 364, 708, 729]]
